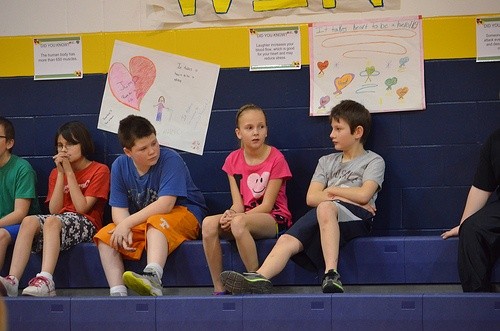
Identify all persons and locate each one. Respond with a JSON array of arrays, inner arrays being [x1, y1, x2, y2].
[[439, 129, 500, 295], [218, 100, 385, 295], [0, 119, 41, 295], [92, 113, 206, 295], [200, 102, 292, 295], [1, 121, 112, 297]]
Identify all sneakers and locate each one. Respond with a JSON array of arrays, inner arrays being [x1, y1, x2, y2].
[[219, 270, 272, 296], [122, 268, 163, 296], [0, 276, 19, 297], [322, 270, 344, 293], [22, 276, 57, 297]]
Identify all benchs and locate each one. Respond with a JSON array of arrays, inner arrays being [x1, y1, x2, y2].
[[0, 58, 500, 331]]
